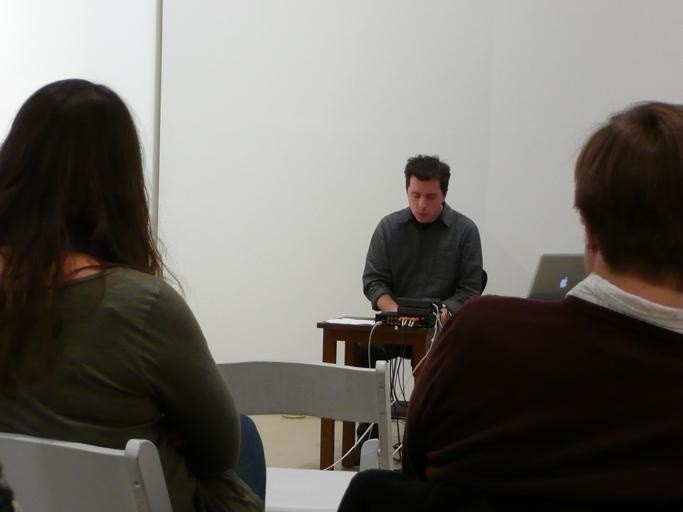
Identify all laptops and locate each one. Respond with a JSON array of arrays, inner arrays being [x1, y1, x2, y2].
[[525, 253, 587, 300]]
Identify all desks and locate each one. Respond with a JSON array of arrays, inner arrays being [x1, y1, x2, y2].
[[315, 316, 441, 469]]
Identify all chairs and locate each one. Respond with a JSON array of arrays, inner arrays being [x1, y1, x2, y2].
[[0, 431, 173, 512], [213, 359, 396, 512]]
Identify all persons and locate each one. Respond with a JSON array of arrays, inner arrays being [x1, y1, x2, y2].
[[350, 153, 488, 464], [399, 101, 682, 499], [0, 80, 265, 511]]
